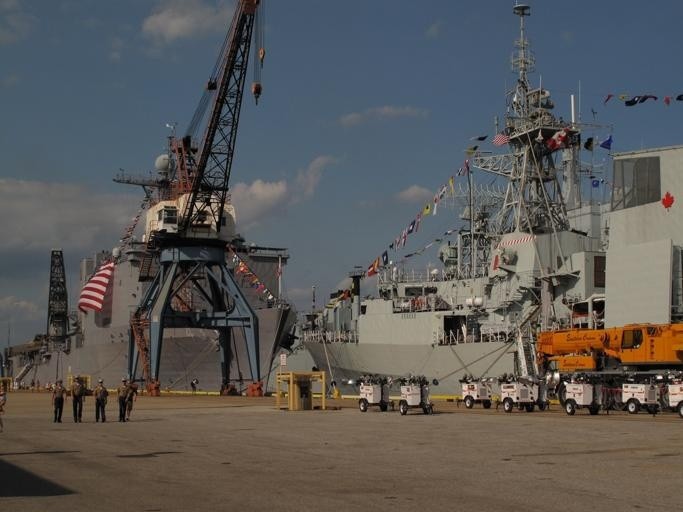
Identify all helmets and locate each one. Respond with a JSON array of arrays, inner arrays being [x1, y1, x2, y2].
[[56, 375, 127, 384]]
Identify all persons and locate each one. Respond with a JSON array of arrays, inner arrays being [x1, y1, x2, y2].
[[116, 377, 130, 422], [70, 375, 86, 423], [50, 379, 67, 423], [93, 378, 109, 423], [126, 379, 138, 420]]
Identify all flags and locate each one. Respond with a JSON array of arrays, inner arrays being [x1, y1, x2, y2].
[[545, 125, 568, 151], [324, 162, 466, 310], [469, 134, 487, 142], [76, 259, 115, 314], [584, 137, 593, 151], [603, 93, 683, 107], [493, 126, 514, 146], [463, 145, 477, 156], [601, 135, 612, 150]]
